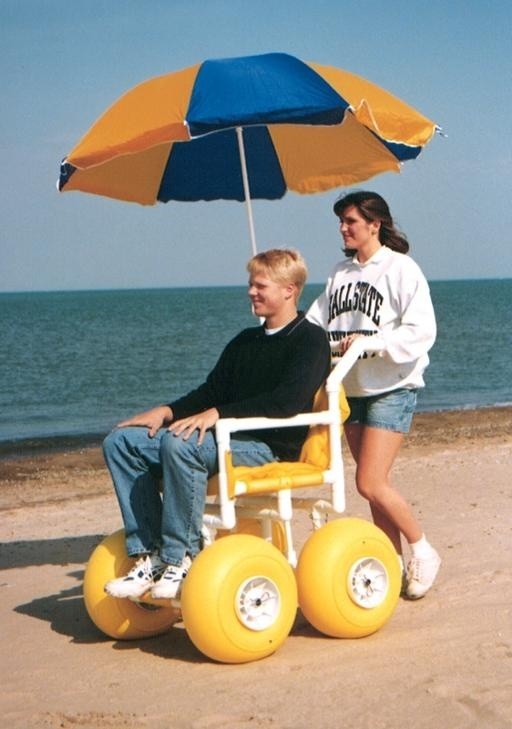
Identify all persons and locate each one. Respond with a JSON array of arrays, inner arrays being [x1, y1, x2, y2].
[[306, 191, 441, 599], [103, 251, 331, 599]]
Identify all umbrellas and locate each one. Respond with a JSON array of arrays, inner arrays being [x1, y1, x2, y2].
[[56, 54, 449, 325]]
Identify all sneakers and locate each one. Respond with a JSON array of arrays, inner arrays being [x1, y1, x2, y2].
[[104, 550, 168, 598], [151, 555, 192, 598], [408, 549, 441, 599]]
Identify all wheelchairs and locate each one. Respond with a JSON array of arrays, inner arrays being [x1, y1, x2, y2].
[[77, 333, 406, 662]]
